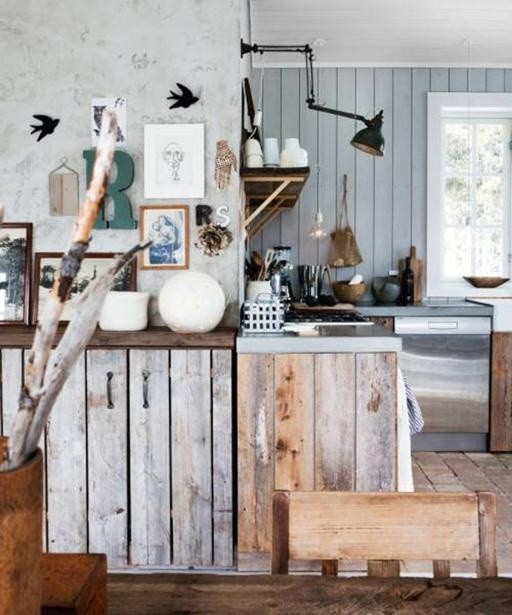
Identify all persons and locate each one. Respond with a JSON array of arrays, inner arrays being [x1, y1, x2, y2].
[[156, 215, 182, 258], [148, 222, 167, 248]]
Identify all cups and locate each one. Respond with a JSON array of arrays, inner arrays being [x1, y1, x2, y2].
[[242, 137, 308, 168]]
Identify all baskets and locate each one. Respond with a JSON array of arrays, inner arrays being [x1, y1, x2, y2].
[[241, 298, 286, 336]]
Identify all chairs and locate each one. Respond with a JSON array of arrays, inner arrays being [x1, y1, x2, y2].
[[271, 489, 497, 578]]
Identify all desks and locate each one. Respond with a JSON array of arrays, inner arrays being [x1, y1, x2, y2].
[[104, 570, 510, 613]]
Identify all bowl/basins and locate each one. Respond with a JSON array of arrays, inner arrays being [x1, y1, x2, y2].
[[332, 281, 368, 304], [463, 276, 510, 288]]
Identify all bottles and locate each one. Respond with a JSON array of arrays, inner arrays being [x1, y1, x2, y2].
[[401, 257, 415, 306]]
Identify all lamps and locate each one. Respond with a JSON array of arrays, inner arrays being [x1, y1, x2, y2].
[[241, 38, 385, 157]]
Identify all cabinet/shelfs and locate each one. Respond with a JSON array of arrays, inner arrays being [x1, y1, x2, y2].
[[0, 345, 236, 577]]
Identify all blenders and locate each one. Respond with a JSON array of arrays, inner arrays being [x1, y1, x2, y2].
[[273, 245, 296, 303]]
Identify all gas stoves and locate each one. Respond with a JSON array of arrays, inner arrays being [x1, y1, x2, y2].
[[283, 309, 375, 325]]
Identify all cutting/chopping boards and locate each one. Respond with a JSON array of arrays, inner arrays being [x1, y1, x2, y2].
[[290, 303, 354, 310]]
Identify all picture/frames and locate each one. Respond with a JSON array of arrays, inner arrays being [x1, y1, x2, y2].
[[1, 206, 190, 326]]
